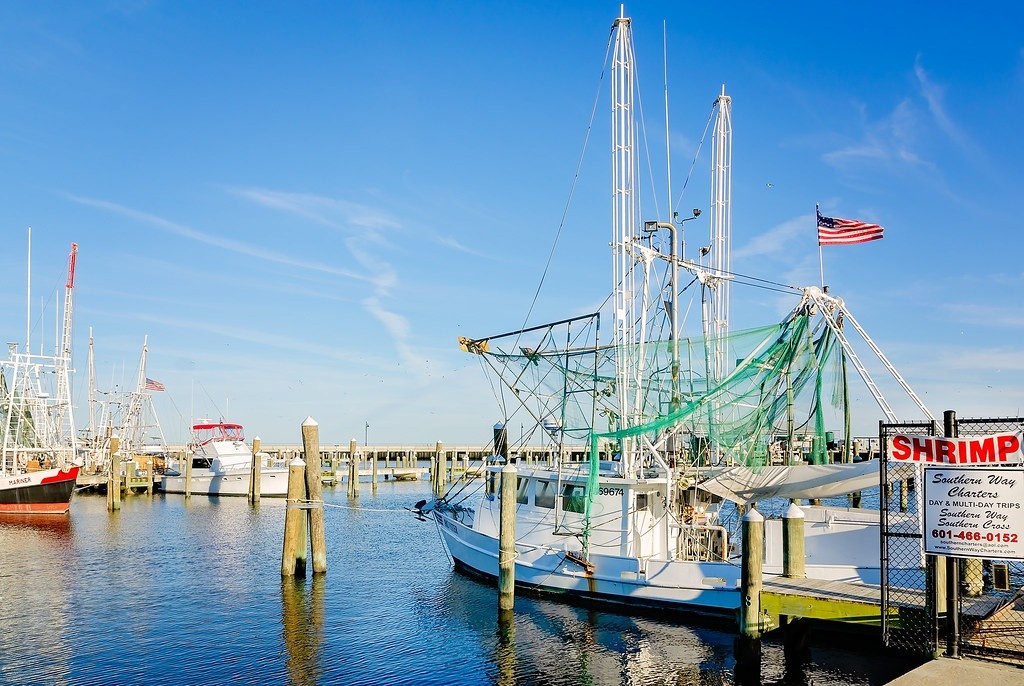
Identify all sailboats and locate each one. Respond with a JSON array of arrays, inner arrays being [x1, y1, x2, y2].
[[0, 224, 186, 518], [402, 0, 1023, 656]]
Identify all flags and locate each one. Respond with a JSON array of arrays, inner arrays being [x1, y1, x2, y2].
[[816, 204, 884, 246], [145, 378, 165, 391]]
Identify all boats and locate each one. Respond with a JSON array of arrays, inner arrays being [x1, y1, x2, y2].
[[158, 378, 289, 499]]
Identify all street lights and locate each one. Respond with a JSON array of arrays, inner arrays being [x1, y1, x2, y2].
[[520, 422, 523, 447], [365, 420, 369, 446]]
